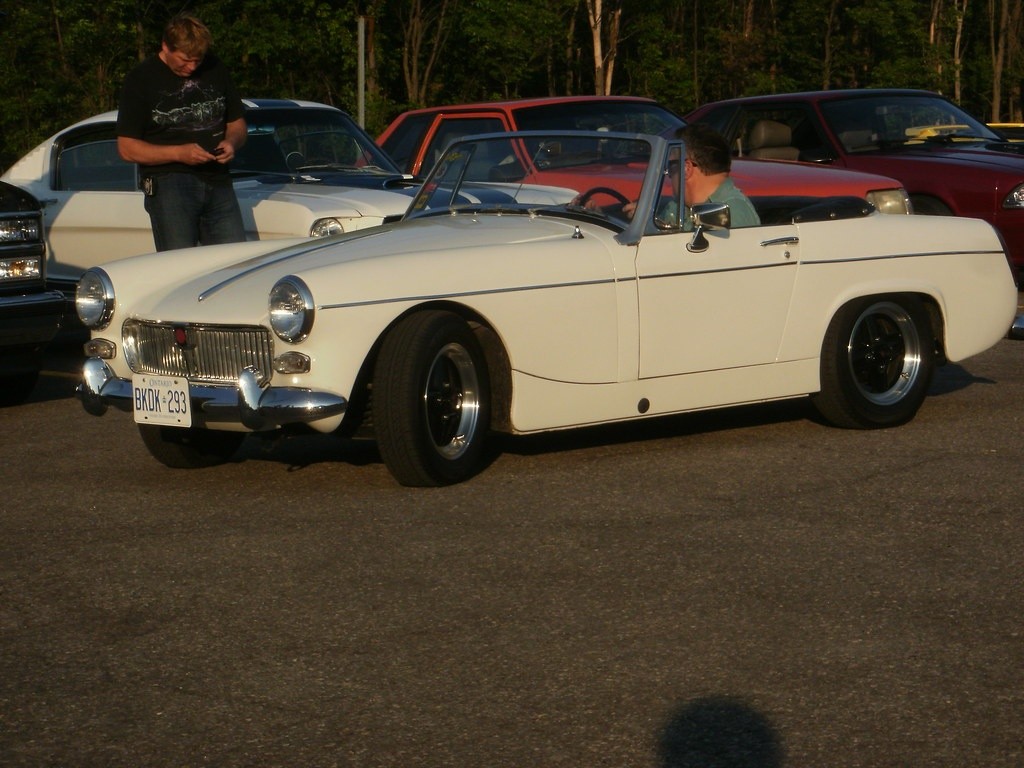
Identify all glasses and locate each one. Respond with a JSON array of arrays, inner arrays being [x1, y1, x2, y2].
[[667, 159, 697, 178]]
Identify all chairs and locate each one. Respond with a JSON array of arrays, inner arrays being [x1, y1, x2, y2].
[[838, 130, 880, 153], [534, 139, 581, 167], [748, 119, 800, 160], [436, 132, 506, 182]]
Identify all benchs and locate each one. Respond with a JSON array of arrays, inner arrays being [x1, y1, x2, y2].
[[601, 195, 874, 225]]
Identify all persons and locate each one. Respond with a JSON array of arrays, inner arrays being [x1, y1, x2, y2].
[[116, 14, 246, 253], [623, 123, 759, 229]]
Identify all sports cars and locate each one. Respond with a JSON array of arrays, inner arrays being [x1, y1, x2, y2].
[[74, 129, 1021, 489]]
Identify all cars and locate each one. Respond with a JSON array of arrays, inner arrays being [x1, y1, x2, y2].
[[1, 180, 70, 348], [639, 87, 1024, 281], [349, 94, 914, 218], [0, 97, 585, 300]]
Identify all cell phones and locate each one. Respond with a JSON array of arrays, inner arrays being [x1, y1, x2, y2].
[[209, 148, 225, 155]]
